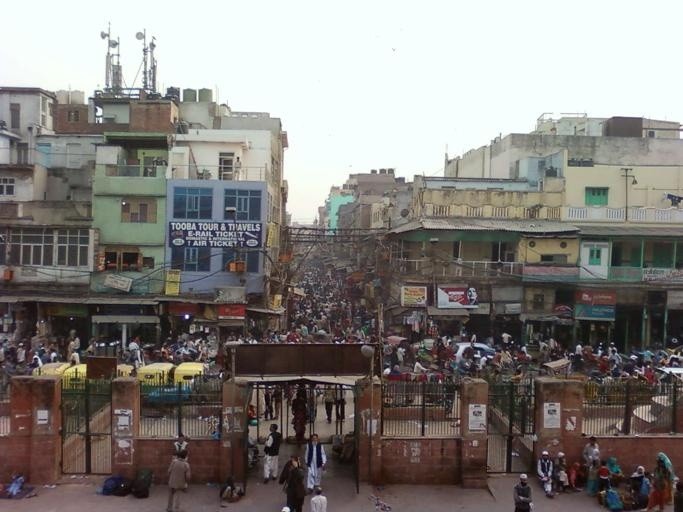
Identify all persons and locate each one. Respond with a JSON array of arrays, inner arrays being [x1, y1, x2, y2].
[[172, 117, 192, 135], [233, 156, 242, 181]]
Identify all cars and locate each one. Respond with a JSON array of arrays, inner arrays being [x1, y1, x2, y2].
[[450, 342, 495, 363]]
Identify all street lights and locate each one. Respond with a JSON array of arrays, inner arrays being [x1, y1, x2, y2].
[[361, 345, 375, 483], [225, 340, 241, 488]]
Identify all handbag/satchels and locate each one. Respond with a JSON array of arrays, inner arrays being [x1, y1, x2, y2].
[[131, 479, 148, 498], [101, 476, 130, 497]]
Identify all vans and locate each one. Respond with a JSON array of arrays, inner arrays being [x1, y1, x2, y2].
[[32, 362, 207, 390]]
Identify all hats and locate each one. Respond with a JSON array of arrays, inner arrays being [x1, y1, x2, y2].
[[519, 473, 527, 478], [542, 450, 564, 457]]
